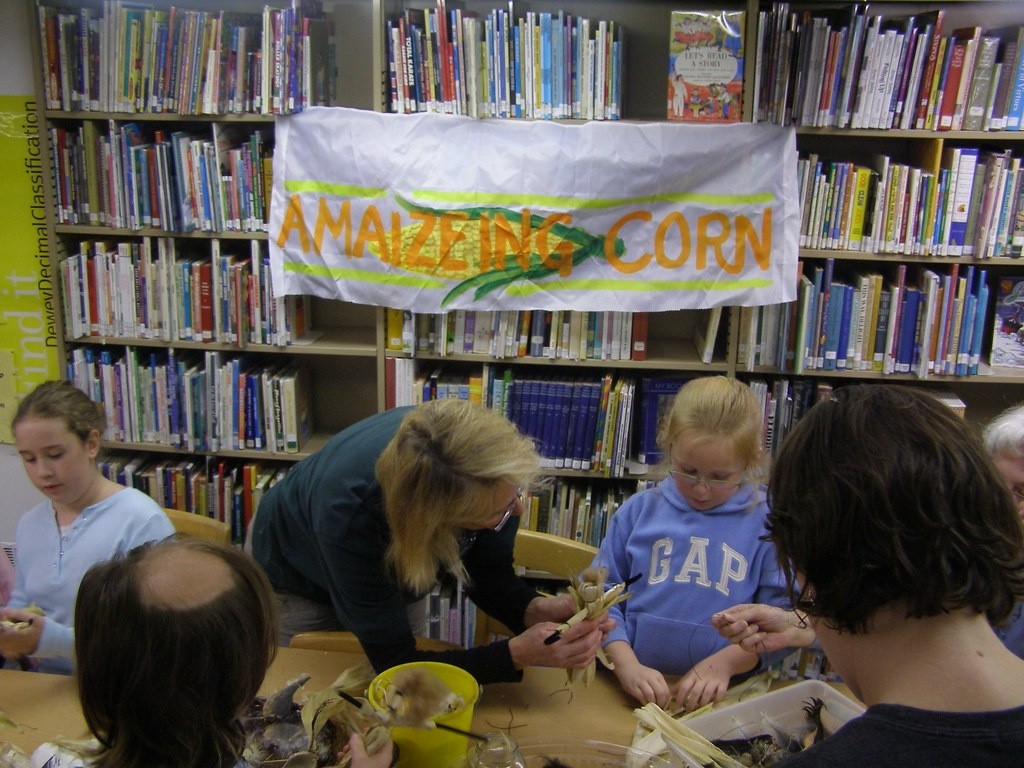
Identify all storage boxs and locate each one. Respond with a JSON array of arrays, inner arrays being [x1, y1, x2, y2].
[[661, 679, 865, 768]]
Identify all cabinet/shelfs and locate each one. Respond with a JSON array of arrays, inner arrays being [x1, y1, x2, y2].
[[29, 0, 1024, 544]]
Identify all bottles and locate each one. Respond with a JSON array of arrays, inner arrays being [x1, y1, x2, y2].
[[467, 731, 527, 768]]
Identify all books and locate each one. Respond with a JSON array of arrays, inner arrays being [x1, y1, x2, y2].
[[383, 1, 625, 119], [58, 237, 312, 349], [512, 479, 661, 572], [385, 357, 693, 478], [667, 0, 1023, 131], [693, 258, 1024, 380], [98, 456, 288, 553], [383, 306, 649, 361], [795, 144, 1024, 261], [747, 380, 965, 488], [419, 572, 509, 651], [66, 349, 313, 453], [31, 0, 337, 117], [50, 117, 273, 234]]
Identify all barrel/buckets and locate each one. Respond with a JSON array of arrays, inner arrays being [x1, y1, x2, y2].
[[368, 661, 480, 768]]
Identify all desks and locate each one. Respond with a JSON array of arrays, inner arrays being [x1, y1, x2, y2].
[[0, 647, 865, 768]]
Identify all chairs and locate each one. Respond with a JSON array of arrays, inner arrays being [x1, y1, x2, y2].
[[161, 507, 232, 548], [288, 632, 465, 655], [472, 529, 599, 647]]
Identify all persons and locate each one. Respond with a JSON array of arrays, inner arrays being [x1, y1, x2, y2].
[[578, 374, 800, 711], [244, 398, 617, 685], [711, 403, 1023, 658], [29, 531, 394, 768], [0, 378, 175, 675], [753, 384, 1023, 768]]
